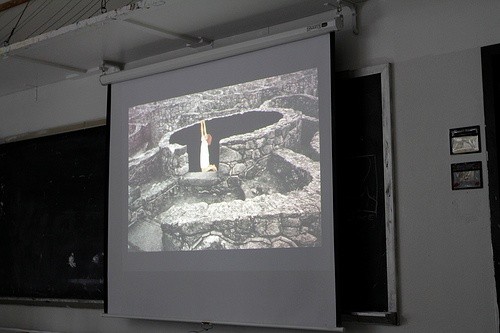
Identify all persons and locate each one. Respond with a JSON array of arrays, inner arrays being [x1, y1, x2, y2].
[[199, 120, 218, 172]]
